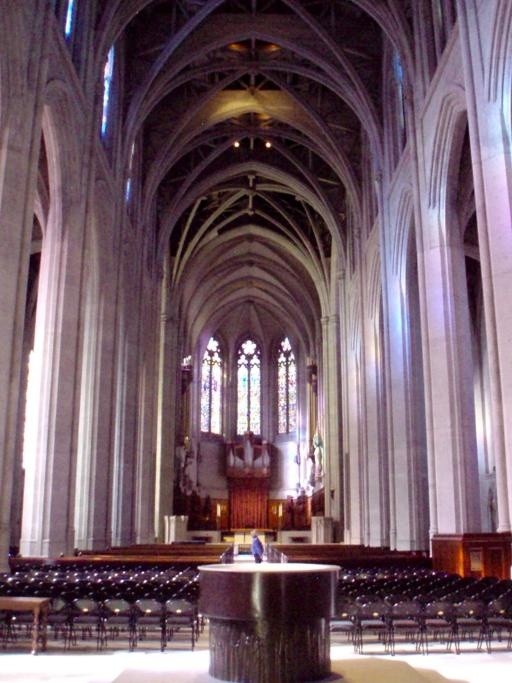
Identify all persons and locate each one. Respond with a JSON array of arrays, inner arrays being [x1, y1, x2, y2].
[[249, 528, 264, 562]]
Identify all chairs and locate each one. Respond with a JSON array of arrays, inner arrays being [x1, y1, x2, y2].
[[263, 543, 511, 656], [1, 544, 230, 656]]
